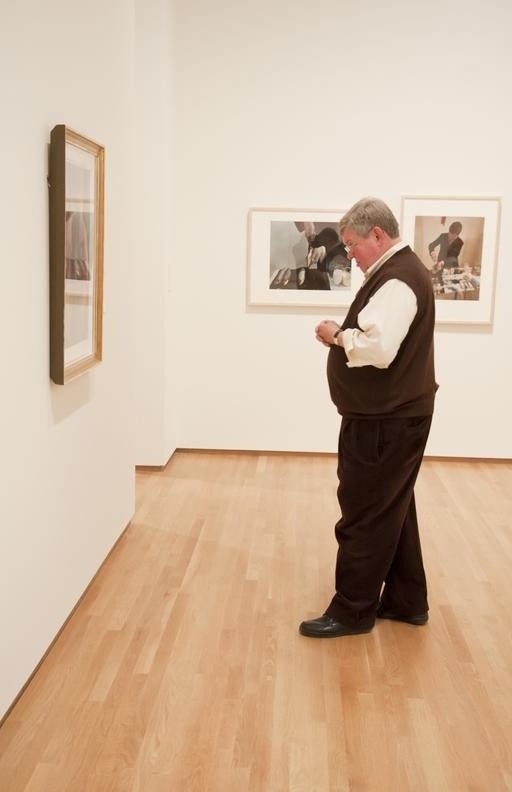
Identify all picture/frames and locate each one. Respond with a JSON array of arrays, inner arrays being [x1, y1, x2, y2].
[[246, 197, 504, 333], [47, 121, 109, 386]]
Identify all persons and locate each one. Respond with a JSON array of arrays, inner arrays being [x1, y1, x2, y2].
[[428, 221, 462, 272], [298, 198, 462, 637]]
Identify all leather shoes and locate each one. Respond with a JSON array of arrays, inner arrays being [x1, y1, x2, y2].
[[374, 602, 428, 624], [300, 613, 374, 636]]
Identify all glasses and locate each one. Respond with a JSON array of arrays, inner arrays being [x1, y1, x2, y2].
[[343, 227, 374, 253]]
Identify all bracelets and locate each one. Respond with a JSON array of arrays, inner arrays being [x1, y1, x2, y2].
[[333, 330, 341, 346]]
